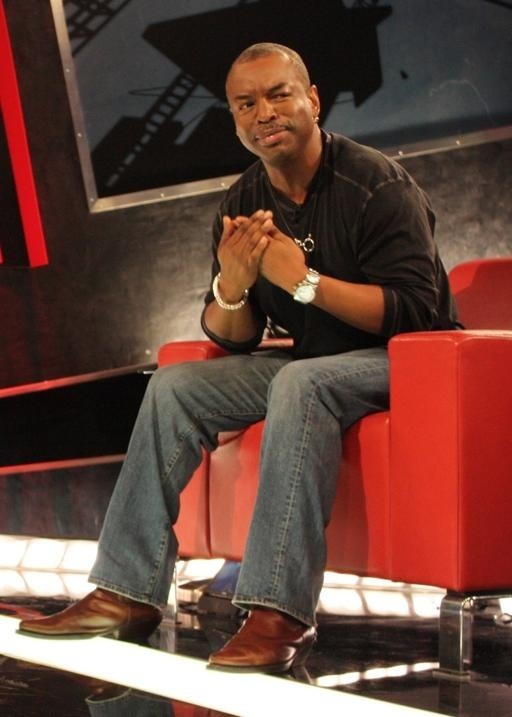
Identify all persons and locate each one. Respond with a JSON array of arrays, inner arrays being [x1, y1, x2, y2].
[[15, 43, 466, 675], [70, 674, 174, 717]]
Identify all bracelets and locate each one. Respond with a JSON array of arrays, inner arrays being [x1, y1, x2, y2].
[[212, 271, 249, 310]]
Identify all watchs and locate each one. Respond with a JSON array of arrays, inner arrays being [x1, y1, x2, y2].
[[292, 267, 320, 305]]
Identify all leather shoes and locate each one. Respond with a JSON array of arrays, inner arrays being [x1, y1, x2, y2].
[[205, 604, 319, 674], [15, 587, 163, 642]]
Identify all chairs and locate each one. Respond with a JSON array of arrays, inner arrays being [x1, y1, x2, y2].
[[148, 257, 512, 678]]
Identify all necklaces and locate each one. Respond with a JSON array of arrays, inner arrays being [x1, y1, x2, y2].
[[267, 178, 318, 253]]
